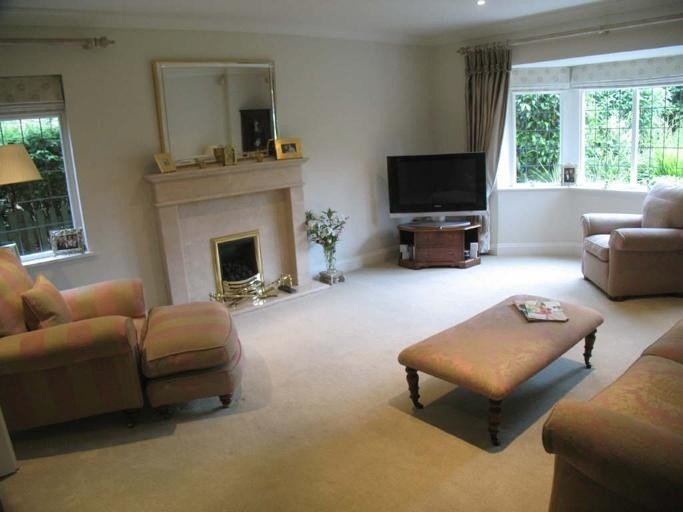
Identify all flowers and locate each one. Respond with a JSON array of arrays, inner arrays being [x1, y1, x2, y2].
[[304, 208, 351, 246]]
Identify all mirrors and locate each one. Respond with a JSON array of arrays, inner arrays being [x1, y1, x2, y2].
[[153, 57, 277, 166]]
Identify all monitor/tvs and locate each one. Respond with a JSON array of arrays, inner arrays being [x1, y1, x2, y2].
[[386, 151, 487, 228]]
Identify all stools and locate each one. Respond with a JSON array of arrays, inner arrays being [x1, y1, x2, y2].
[[139, 301, 244, 419]]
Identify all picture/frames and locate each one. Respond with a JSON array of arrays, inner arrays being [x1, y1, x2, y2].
[[273, 137, 302, 160], [560, 164, 577, 186], [153, 152, 177, 173], [50, 227, 85, 255]]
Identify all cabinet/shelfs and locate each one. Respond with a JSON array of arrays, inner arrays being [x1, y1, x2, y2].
[[395, 221, 482, 269], [236, 108, 271, 155]]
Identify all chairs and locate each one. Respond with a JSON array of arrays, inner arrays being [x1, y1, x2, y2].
[[579, 178, 682, 302], [0, 244, 145, 435]]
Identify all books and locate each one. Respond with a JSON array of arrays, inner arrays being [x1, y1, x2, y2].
[[511, 299, 569, 322], [525, 301, 567, 321]]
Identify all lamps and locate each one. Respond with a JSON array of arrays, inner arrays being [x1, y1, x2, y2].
[[0, 142, 43, 188]]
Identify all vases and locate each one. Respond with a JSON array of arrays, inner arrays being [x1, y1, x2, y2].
[[323, 249, 337, 274]]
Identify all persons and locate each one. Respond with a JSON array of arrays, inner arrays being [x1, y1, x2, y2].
[[564, 170, 570, 181], [289, 144, 295, 153], [56, 235, 78, 250], [534, 301, 551, 319], [283, 146, 288, 153]]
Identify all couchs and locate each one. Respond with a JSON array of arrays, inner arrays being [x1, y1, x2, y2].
[[542, 318, 682, 509]]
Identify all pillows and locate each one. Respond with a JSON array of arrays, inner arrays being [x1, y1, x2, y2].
[[19, 272, 72, 335]]
